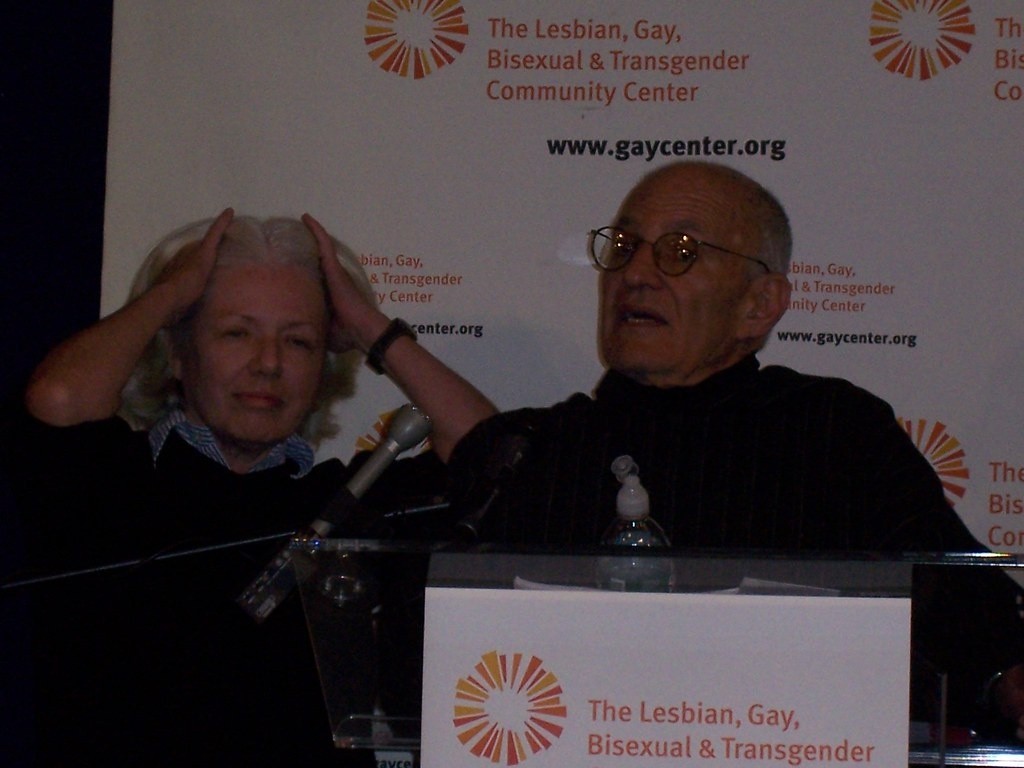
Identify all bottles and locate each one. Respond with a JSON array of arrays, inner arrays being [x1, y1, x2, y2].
[[601, 477, 677, 593]]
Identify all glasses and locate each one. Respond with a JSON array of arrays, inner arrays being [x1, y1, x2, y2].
[[589, 225, 773, 278]]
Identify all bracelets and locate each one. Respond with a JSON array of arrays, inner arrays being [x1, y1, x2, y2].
[[365, 317, 418, 373]]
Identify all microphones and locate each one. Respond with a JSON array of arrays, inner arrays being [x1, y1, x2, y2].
[[238, 404, 434, 624]]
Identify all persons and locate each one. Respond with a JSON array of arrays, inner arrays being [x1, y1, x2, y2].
[[441, 160, 1024, 743], [1, 208, 502, 767]]
